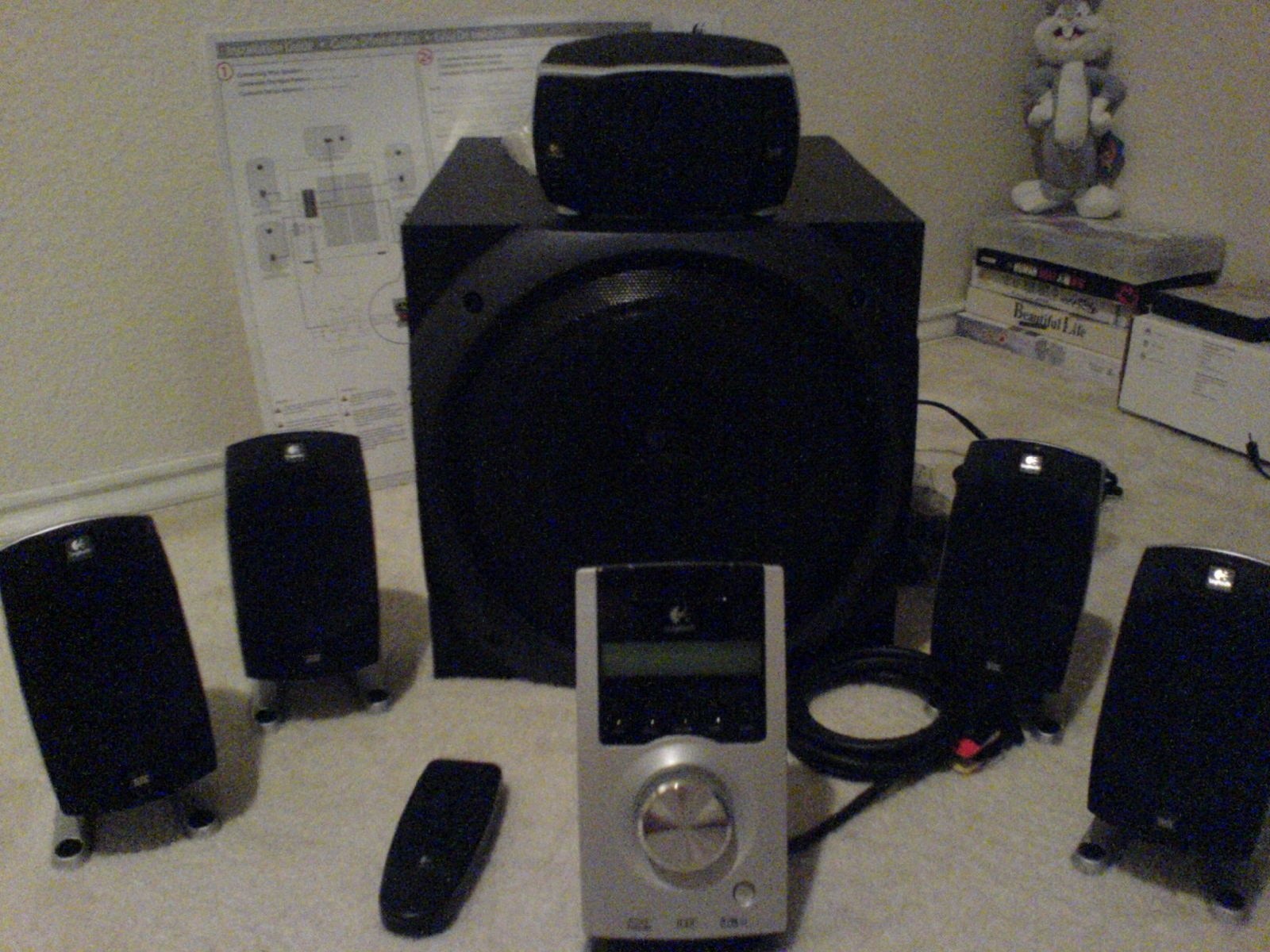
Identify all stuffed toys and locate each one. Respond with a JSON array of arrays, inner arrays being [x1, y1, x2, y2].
[[1012, 0, 1128, 219]]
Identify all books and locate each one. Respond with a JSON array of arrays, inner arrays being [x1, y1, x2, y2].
[[956, 207, 1225, 382]]
